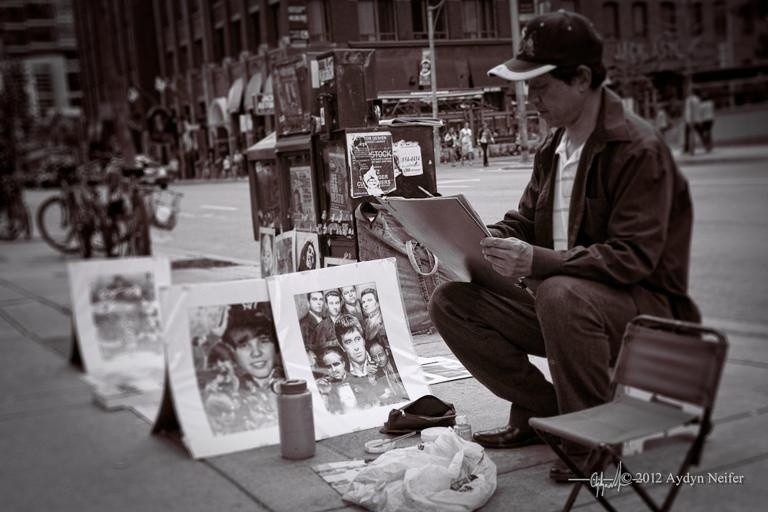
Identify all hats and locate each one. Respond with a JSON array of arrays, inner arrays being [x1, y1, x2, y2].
[[488, 10, 604, 81]]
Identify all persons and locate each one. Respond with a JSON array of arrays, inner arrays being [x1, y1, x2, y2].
[[313, 290, 350, 351], [361, 288, 387, 342], [426, 9, 694, 481], [205, 391, 240, 437], [316, 345, 375, 414], [335, 314, 370, 385], [298, 290, 325, 351], [259, 234, 274, 276], [223, 149, 243, 180], [299, 240, 318, 274], [206, 343, 246, 400], [339, 284, 365, 327], [228, 309, 284, 393], [681, 84, 715, 153], [443, 124, 456, 167], [459, 119, 474, 165], [478, 118, 494, 168], [369, 338, 407, 403], [653, 99, 669, 136]]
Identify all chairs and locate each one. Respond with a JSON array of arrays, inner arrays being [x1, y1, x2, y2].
[[527, 314, 729, 512]]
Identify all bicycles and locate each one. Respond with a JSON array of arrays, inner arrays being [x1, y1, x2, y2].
[[36, 161, 93, 258], [111, 158, 155, 256], [83, 161, 113, 254]]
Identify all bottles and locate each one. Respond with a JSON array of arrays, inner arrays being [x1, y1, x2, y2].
[[453, 414, 473, 442], [270, 378, 317, 461]]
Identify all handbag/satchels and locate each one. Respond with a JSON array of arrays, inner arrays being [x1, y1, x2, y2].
[[446, 132, 453, 147], [381, 395, 456, 435]]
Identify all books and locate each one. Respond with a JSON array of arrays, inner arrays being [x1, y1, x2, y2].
[[377, 191, 541, 305]]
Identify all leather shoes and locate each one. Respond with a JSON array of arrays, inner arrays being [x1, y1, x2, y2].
[[473, 423, 561, 449], [549, 442, 622, 482]]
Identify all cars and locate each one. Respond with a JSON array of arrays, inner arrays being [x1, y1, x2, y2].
[[137, 159, 172, 191]]
